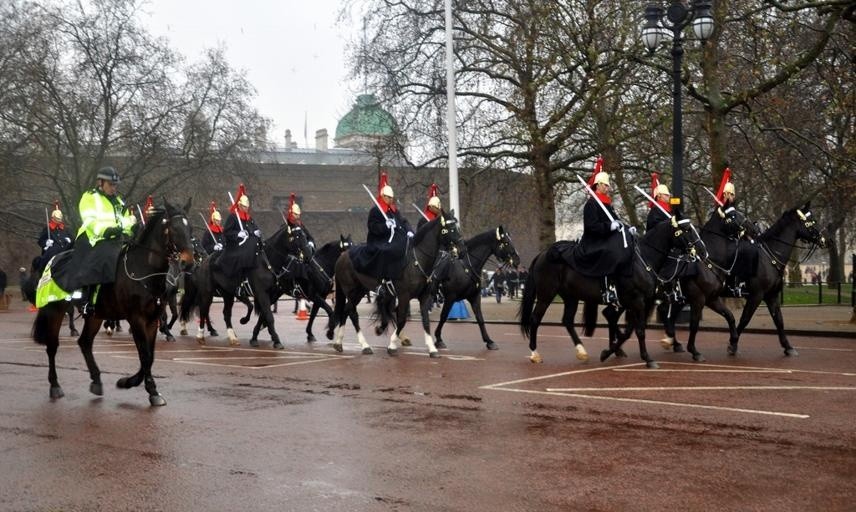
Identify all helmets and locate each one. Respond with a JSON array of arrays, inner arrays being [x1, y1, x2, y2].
[[654, 185, 672, 200], [96, 167, 119, 184], [380, 186, 393, 198], [723, 182, 736, 196], [428, 195, 442, 210], [211, 211, 222, 222], [49, 209, 64, 219], [236, 194, 250, 206], [289, 204, 302, 215], [594, 172, 613, 192]]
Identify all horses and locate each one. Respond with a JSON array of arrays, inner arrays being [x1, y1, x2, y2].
[[59, 222, 356, 349], [30, 195, 193, 407], [365, 224, 521, 350], [581, 198, 760, 363], [656, 200, 832, 358], [325, 207, 465, 358], [514, 214, 709, 369]]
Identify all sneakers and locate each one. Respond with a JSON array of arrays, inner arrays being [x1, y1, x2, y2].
[[731, 289, 750, 297], [664, 294, 684, 303], [601, 294, 619, 304], [78, 304, 95, 312]]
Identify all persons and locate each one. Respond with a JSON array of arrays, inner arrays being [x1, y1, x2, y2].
[[214, 194, 263, 297], [33, 209, 74, 292], [647, 183, 684, 231], [202, 210, 225, 255], [478, 264, 530, 304], [69, 166, 138, 316], [0, 269, 8, 310], [288, 202, 316, 250], [368, 184, 416, 298], [416, 196, 442, 230], [700, 179, 761, 297], [580, 170, 633, 307], [18, 266, 30, 301], [144, 205, 157, 220]]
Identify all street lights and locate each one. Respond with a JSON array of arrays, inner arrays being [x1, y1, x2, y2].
[[642, 0, 715, 217], [373, 141, 386, 182]]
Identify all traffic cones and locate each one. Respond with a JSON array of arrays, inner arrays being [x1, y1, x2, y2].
[[295, 298, 310, 320]]
